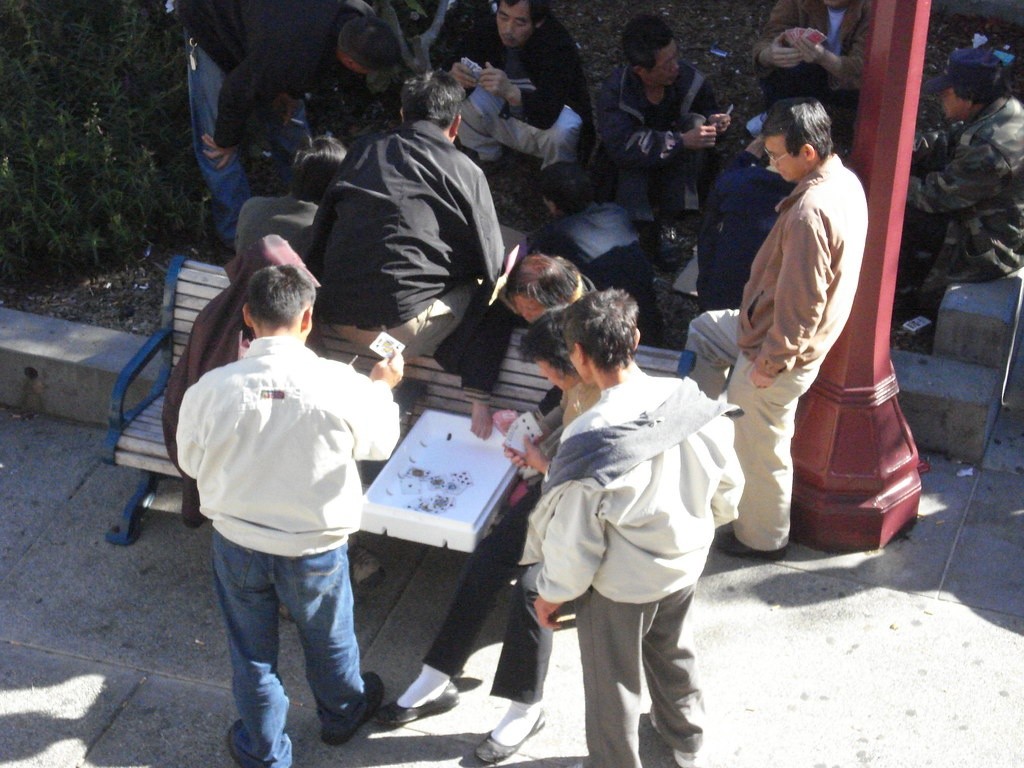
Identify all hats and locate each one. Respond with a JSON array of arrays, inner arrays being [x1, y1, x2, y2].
[[923, 48, 1004, 92]]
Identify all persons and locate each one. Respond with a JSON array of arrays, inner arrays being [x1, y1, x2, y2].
[[684, 97, 868, 559], [304, 63, 505, 361], [160, 236, 389, 622], [515, 285, 745, 767], [445, 0, 595, 183], [234, 133, 349, 263], [535, 156, 665, 348], [594, 16, 732, 257], [695, 0, 870, 313], [899, 44, 1024, 299], [433, 250, 599, 439], [172, 0, 399, 253], [176, 267, 405, 768], [371, 311, 600, 763]]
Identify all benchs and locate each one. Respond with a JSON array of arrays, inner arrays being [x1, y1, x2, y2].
[[101, 257, 695, 548]]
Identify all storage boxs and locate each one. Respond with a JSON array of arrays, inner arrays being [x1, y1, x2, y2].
[[360, 408, 519, 553]]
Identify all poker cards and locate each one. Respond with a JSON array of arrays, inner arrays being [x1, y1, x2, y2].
[[711, 103, 736, 127], [400, 464, 475, 515], [460, 57, 484, 87], [784, 25, 829, 48], [490, 408, 519, 437], [369, 331, 407, 360], [501, 411, 544, 453]]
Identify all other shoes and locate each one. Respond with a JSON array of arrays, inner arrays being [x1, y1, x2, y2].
[[476, 709, 546, 763], [716, 528, 786, 562], [375, 679, 460, 723], [227, 718, 244, 768], [321, 673, 385, 744], [649, 209, 681, 269]]
